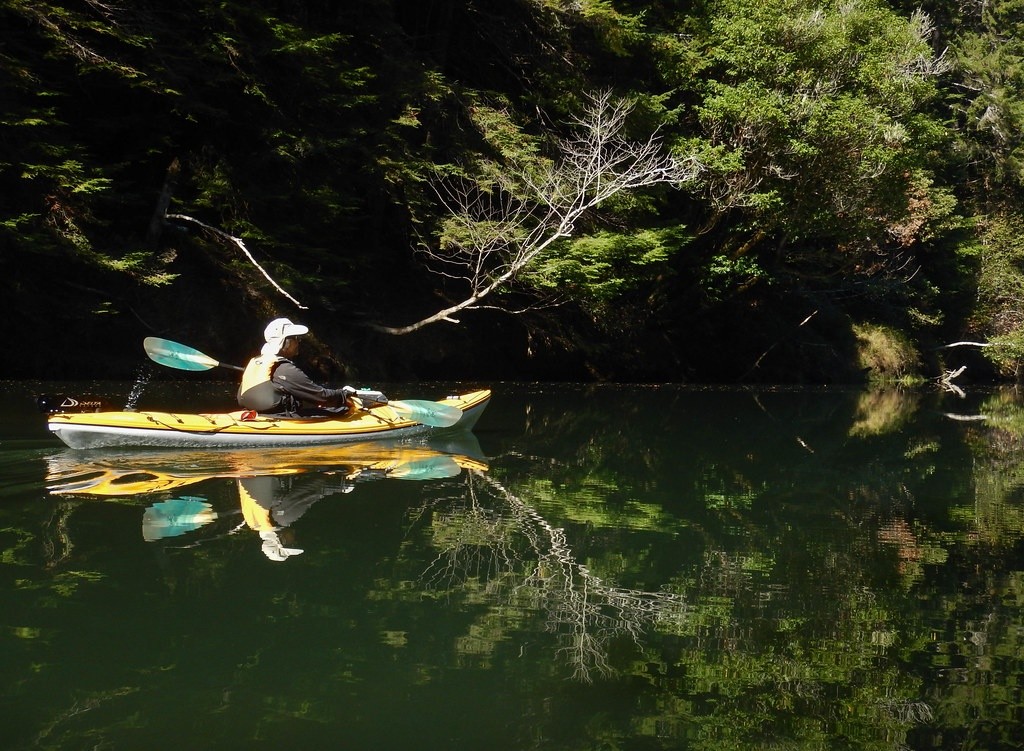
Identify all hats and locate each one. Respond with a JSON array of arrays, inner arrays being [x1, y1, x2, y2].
[[259, 317, 309, 355]]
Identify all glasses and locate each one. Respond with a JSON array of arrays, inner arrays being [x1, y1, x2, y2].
[[285, 335, 300, 341]]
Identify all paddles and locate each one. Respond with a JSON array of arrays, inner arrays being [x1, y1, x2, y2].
[[142, 337, 464, 429], [142, 455, 462, 543]]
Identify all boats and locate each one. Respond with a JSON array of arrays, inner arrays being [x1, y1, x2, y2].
[[47, 388, 493, 450]]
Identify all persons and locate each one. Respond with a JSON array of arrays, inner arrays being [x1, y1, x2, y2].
[[236, 319, 361, 414], [234, 476, 354, 560]]
